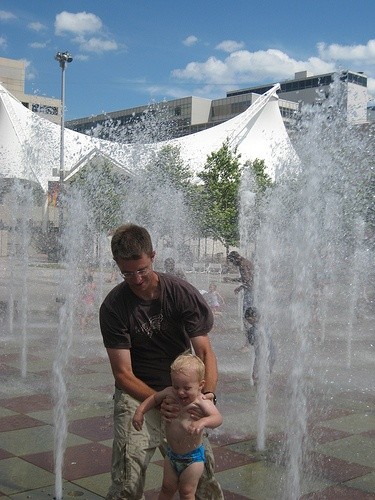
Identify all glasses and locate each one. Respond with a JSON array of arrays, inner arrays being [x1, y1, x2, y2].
[[119, 262, 151, 279]]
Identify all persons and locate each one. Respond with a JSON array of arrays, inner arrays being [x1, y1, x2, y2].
[[81, 225, 118, 333], [164, 258, 187, 281], [99, 223, 224, 500], [204, 283, 225, 317], [242, 307, 277, 402], [132, 354, 223, 500], [222, 251, 257, 354]]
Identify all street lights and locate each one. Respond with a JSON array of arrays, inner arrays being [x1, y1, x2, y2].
[[54, 50, 75, 231]]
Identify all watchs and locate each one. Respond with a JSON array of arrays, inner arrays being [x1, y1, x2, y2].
[[202, 392, 217, 405]]
